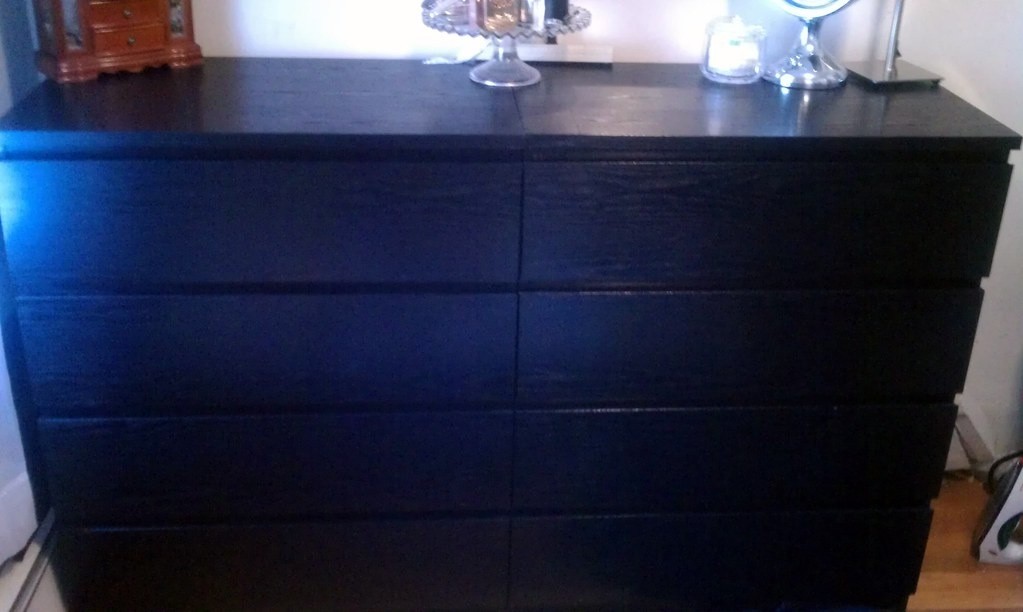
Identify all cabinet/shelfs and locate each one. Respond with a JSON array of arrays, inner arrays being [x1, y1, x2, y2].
[[0, 55, 1023, 612]]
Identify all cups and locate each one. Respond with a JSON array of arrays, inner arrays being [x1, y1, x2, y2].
[[701, 17, 764, 84]]
